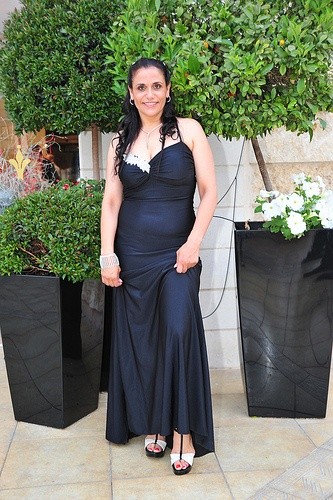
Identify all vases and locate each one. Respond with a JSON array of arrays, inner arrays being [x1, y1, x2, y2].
[[233, 221, 333, 419]]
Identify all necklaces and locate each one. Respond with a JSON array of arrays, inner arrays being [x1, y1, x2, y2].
[[140, 122, 163, 136]]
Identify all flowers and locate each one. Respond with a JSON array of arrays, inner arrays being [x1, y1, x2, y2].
[[254, 172, 333, 239]]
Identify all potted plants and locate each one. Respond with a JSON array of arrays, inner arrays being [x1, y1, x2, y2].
[[1, 178, 107, 430]]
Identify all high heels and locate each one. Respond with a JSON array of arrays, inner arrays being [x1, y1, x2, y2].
[[170, 428, 195, 475], [144, 434, 167, 457]]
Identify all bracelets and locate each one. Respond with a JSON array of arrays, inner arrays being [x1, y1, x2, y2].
[[100, 253, 120, 268]]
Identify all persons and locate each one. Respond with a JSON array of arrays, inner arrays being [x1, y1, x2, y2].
[[0, 161, 28, 215], [45, 154, 62, 178], [99, 58, 218, 476], [23, 145, 59, 194]]
